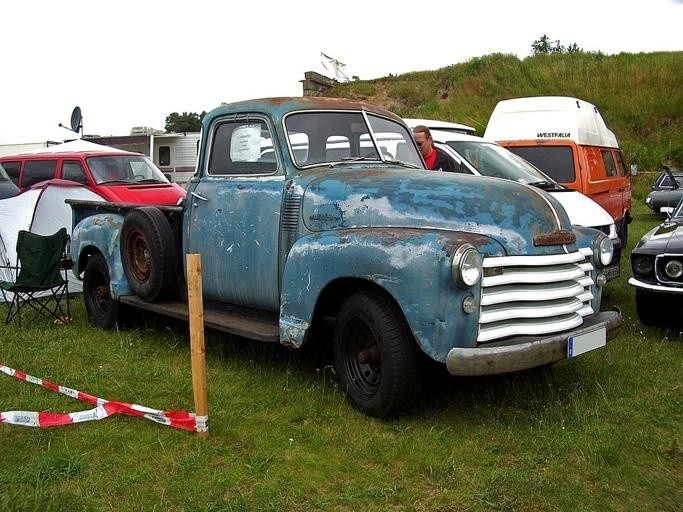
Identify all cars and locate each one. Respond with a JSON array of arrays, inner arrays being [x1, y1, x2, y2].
[[627, 166, 683, 325]]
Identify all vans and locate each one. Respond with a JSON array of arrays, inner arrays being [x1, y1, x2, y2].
[[0, 152, 187, 209], [258, 95, 622, 281], [482, 96, 639, 237]]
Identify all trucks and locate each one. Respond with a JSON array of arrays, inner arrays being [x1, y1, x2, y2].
[[64, 97, 623, 418]]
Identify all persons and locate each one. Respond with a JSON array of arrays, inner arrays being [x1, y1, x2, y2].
[[629, 158, 638, 176], [411, 124, 450, 172]]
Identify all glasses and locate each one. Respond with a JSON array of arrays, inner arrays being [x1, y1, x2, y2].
[[417, 142, 423, 146]]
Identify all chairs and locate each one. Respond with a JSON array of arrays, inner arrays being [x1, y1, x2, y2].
[[0, 227, 70, 329]]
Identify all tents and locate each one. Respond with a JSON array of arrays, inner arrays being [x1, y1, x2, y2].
[[0, 176, 109, 304]]
